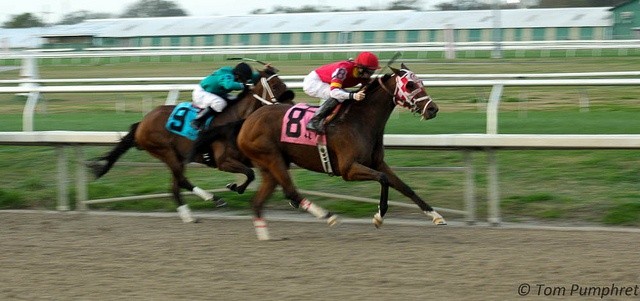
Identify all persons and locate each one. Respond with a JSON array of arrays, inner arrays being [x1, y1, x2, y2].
[[191, 62, 271, 129], [303, 51, 381, 135]]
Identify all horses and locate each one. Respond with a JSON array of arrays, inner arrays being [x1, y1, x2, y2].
[[188, 62, 447, 239], [83, 65, 302, 223]]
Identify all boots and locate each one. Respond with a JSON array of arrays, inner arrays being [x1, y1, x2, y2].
[[191, 105, 217, 129], [305, 96, 340, 133]]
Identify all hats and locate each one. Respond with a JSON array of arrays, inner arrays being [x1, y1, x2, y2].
[[355, 51, 381, 68], [234, 62, 252, 76]]
